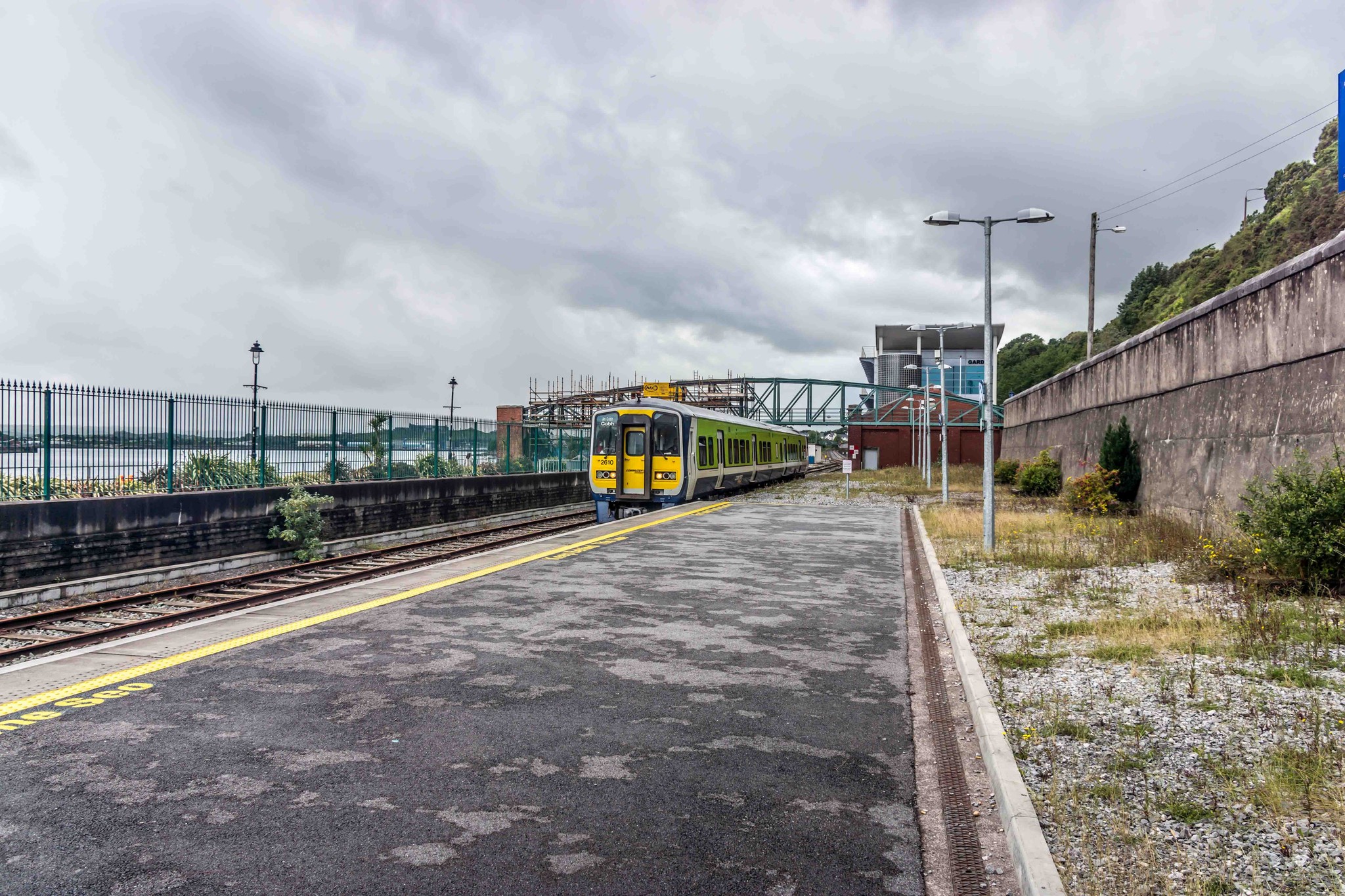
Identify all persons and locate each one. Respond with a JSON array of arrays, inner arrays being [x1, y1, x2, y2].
[[667, 437, 679, 454]]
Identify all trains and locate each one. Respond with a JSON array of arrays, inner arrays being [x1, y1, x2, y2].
[[588, 399, 809, 525]]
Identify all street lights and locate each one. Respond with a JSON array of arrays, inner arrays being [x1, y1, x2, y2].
[[907, 384, 942, 479], [905, 321, 977, 505], [243, 340, 268, 464], [905, 398, 936, 471], [547, 396, 553, 460], [448, 376, 459, 463], [922, 207, 1056, 554], [901, 406, 927, 467], [902, 364, 953, 490], [1086, 211, 1127, 359]]
